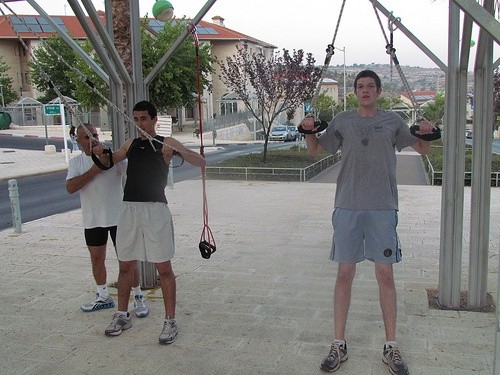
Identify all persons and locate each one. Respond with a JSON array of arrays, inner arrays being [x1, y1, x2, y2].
[[94, 100, 205, 344], [66, 123, 149, 318], [301, 70, 436, 375]]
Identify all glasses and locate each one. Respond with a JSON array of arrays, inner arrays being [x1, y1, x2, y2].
[[78, 134, 99, 139]]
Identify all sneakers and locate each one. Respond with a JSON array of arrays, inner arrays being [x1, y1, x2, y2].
[[382, 344, 409, 375], [81, 293, 115, 312], [159, 316, 178, 343], [105, 312, 133, 336], [320, 340, 348, 372], [134, 293, 149, 318]]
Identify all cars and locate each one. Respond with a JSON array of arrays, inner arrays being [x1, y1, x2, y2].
[[268, 125, 327, 142]]
